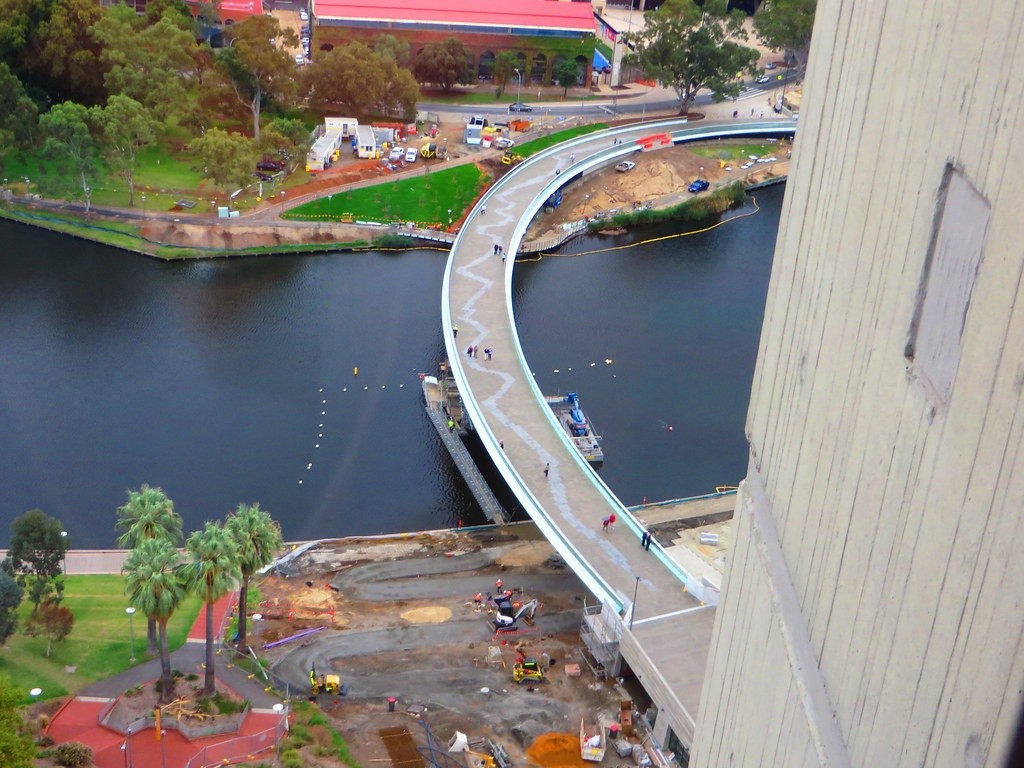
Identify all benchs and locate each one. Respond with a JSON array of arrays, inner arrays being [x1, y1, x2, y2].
[[391, 222, 405, 225], [356, 220, 382, 226], [341, 219, 353, 222]]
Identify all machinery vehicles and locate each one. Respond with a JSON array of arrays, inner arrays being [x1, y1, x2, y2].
[[308, 667, 347, 696], [514, 658, 544, 684], [484, 596, 538, 633]]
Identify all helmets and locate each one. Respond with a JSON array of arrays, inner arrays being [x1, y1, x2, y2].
[[504, 590, 506, 594], [479, 593, 482, 596], [498, 579, 500, 582]]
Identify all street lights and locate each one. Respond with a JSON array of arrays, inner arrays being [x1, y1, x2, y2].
[[766, 63, 777, 70], [61, 531, 68, 578], [126, 608, 136, 662], [514, 68, 521, 113]]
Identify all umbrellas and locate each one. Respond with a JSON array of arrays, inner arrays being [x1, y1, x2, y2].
[[602, 514, 610, 522]]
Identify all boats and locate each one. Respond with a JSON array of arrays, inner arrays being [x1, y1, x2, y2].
[[545, 394, 605, 462], [422, 375, 452, 405], [439, 377, 468, 422]]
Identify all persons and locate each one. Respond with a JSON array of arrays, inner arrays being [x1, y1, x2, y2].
[[313, 674, 323, 695], [453, 323, 493, 361], [730, 86, 794, 160], [500, 440, 504, 449], [601, 513, 616, 532], [613, 136, 623, 144], [481, 203, 507, 261], [555, 153, 576, 174], [642, 530, 651, 550], [448, 418, 456, 434], [545, 462, 549, 476], [474, 578, 520, 607]]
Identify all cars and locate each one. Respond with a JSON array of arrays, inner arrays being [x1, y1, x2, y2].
[[688, 179, 709, 193], [498, 140, 515, 147], [509, 102, 532, 112], [614, 160, 635, 173], [756, 75, 769, 83], [404, 148, 418, 163], [254, 170, 273, 182], [389, 147, 404, 160], [258, 161, 278, 172]]
